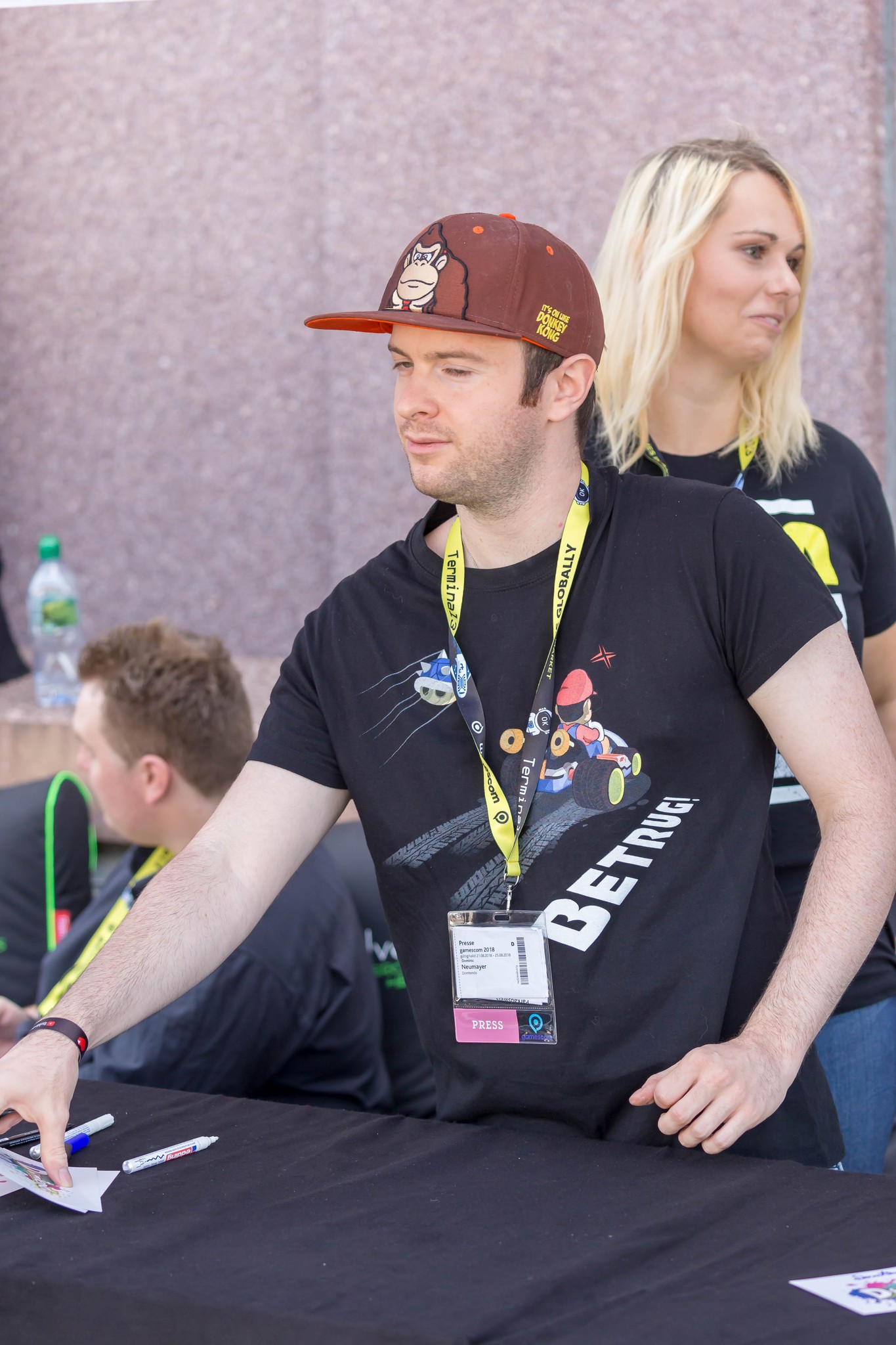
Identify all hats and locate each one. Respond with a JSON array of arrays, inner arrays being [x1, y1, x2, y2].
[[304, 213, 605, 370]]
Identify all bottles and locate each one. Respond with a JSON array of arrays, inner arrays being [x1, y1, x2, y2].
[[27, 534, 81, 707]]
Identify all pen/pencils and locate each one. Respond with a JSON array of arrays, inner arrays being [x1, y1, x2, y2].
[[122, 1133, 219, 1173], [0, 1123, 75, 1146], [29, 1113, 114, 1160]]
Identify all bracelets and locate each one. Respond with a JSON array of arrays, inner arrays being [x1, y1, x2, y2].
[[24, 1017, 89, 1066]]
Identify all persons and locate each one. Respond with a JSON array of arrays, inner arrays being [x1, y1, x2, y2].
[[582, 131, 896, 1172], [0, 621, 390, 1115], [0, 212, 896, 1190]]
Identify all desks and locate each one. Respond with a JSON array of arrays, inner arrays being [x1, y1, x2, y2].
[[0, 659, 359, 846], [0, 1080, 896, 1345]]
